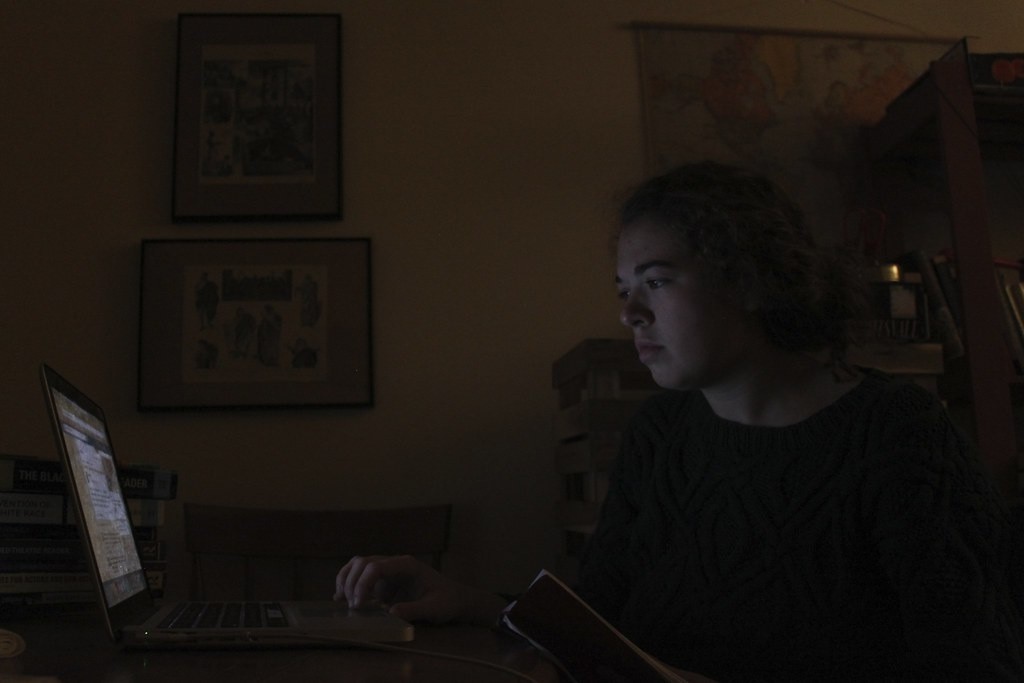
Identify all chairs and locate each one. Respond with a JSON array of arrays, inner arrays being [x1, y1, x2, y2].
[[184, 500, 454, 612]]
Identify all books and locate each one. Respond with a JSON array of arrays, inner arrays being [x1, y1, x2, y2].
[[1, 593, 99, 603], [0, 560, 155, 573], [0, 524, 160, 540], [0, 541, 161, 559], [0, 493, 167, 527], [881, 247, 1024, 378], [0, 574, 164, 593], [0, 454, 177, 500]]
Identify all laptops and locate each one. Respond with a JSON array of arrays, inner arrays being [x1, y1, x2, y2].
[[38, 361, 415, 647]]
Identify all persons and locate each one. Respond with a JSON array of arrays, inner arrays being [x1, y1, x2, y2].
[[331, 158, 1024, 683]]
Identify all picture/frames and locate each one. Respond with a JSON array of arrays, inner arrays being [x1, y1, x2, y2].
[[138, 235, 377, 408], [170, 12, 345, 222]]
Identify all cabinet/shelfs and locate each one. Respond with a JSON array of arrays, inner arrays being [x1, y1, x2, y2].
[[863, 40, 1024, 496]]
